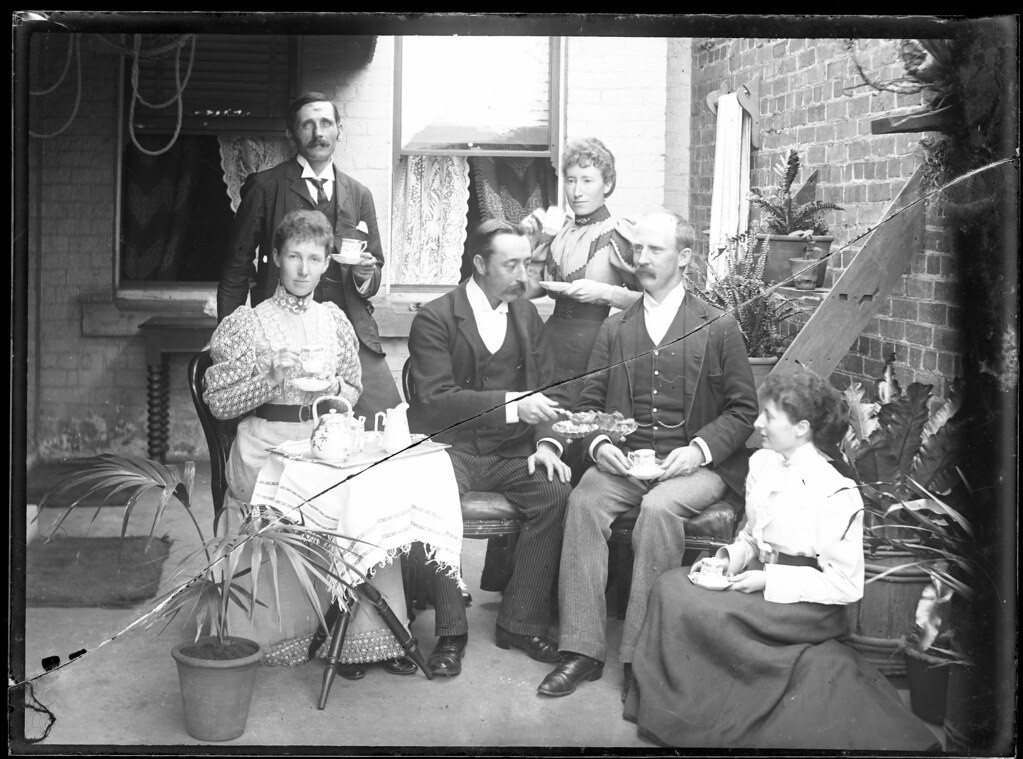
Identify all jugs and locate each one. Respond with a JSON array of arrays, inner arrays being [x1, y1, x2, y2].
[[374, 403, 413, 456]]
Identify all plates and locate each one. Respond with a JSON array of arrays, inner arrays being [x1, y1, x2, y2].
[[331, 254, 367, 264], [291, 377, 330, 391], [536, 280, 570, 291], [627, 465, 665, 480], [687, 572, 734, 591], [552, 421, 600, 438]]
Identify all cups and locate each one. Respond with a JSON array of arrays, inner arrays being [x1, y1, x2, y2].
[[628, 448, 655, 473], [346, 410, 366, 455], [339, 237, 367, 257], [541, 206, 565, 236], [298, 347, 324, 373], [699, 557, 723, 584]]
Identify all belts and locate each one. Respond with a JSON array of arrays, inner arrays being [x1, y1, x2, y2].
[[252, 401, 330, 423], [758, 549, 820, 570]]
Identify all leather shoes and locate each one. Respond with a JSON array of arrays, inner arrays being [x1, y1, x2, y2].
[[428, 631, 468, 675], [495, 625, 565, 663], [622, 662, 633, 701], [461, 592, 472, 606], [337, 660, 365, 679], [538, 651, 604, 696], [378, 655, 418, 674]]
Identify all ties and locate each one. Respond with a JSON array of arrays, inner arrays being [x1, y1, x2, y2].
[[297, 162, 329, 210]]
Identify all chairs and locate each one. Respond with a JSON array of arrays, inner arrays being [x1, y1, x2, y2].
[[187, 348, 249, 542], [608, 483, 745, 567], [401, 354, 521, 612]]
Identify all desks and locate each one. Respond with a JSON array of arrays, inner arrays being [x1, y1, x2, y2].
[[249, 439, 468, 712]]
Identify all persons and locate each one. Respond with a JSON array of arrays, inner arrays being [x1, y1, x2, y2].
[[621, 363, 943, 752], [518, 136, 643, 465], [217, 91, 402, 431], [538, 212, 760, 702], [406, 219, 572, 678], [203, 209, 464, 678]]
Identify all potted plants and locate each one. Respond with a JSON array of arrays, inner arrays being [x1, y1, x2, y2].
[[30, 452, 384, 743], [748, 151, 849, 289], [825, 365, 1006, 759], [680, 229, 804, 391]]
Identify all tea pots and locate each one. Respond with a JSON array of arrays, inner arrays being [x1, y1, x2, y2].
[[310, 396, 353, 462]]
